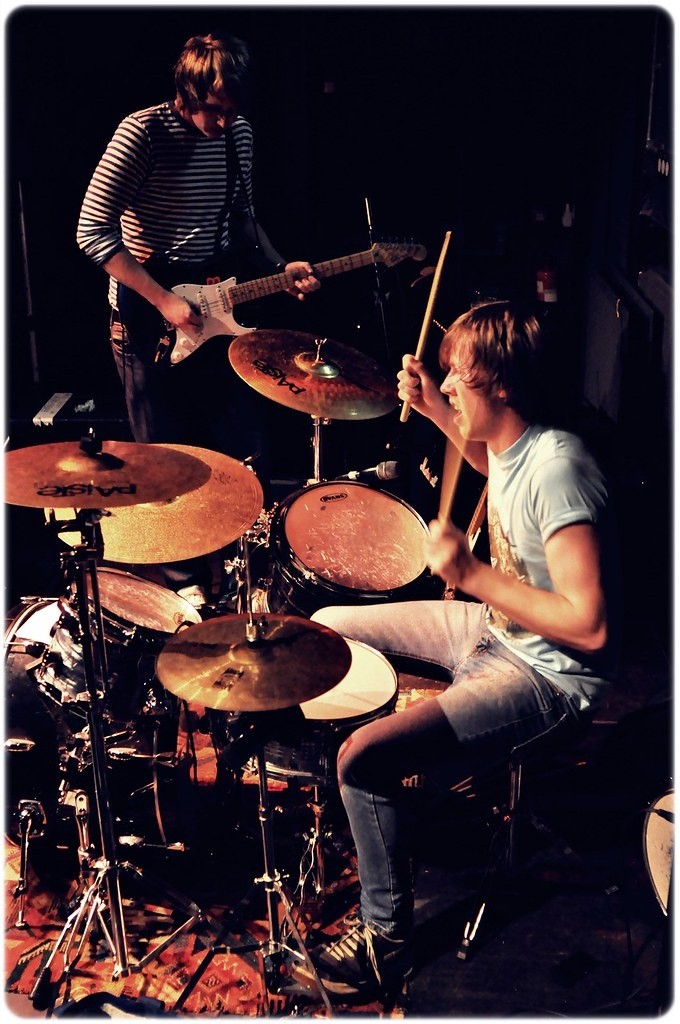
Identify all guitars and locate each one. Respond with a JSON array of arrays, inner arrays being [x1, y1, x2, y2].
[[116, 236, 429, 373]]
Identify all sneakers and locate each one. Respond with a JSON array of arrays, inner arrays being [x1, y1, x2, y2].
[[314, 914, 409, 996]]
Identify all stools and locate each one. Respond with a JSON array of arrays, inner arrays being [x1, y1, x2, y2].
[[445, 696, 621, 965]]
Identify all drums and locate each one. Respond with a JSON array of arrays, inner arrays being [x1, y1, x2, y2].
[[247, 635, 402, 783], [267, 480, 437, 621], [36, 566, 203, 728], [5, 594, 192, 845]]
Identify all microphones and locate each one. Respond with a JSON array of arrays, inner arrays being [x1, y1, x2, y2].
[[334, 460, 400, 483]]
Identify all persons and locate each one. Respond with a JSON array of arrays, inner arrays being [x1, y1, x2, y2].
[[75, 34, 321, 609], [287, 301, 622, 997]]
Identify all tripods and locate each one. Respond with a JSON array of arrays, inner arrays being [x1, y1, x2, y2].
[[27, 502, 360, 1019]]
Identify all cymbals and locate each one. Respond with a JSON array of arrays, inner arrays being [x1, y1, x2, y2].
[[6, 440, 212, 508], [229, 327, 403, 421], [43, 443, 264, 566], [157, 612, 351, 711]]
